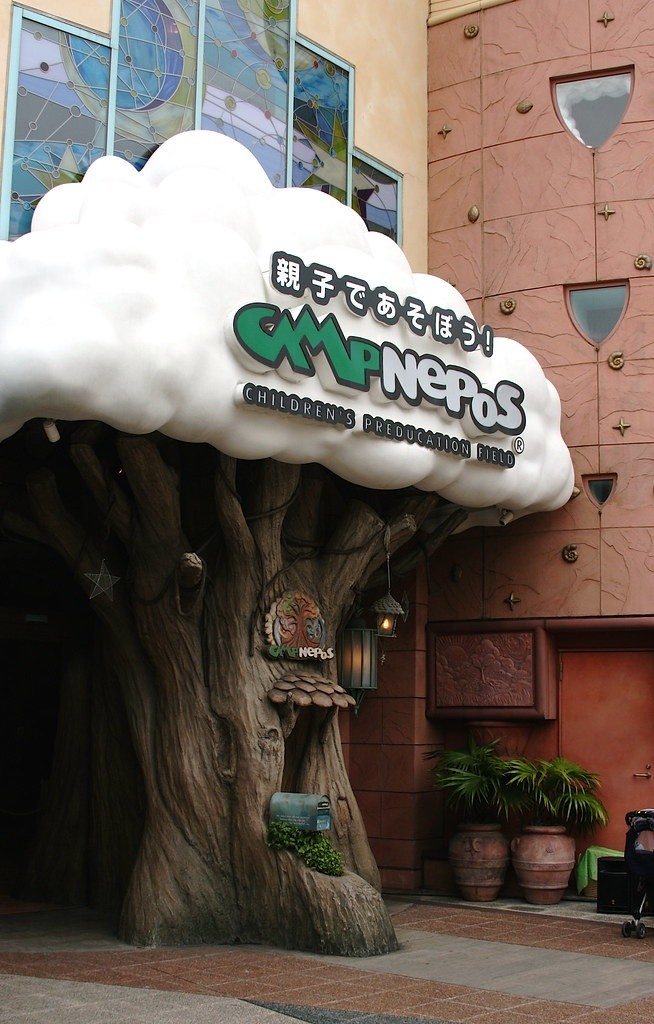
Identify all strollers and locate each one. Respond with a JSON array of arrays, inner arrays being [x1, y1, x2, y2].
[[622, 810, 654, 938]]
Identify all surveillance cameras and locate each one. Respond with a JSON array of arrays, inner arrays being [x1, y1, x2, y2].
[[499, 512, 514, 526], [43, 421, 61, 442]]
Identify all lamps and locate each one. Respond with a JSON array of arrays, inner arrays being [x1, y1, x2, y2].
[[369, 548, 405, 639], [43, 421, 61, 443], [335, 609, 377, 719]]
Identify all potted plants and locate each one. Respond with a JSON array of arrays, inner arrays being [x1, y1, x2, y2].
[[421, 733, 512, 902], [503, 755, 609, 905]]
